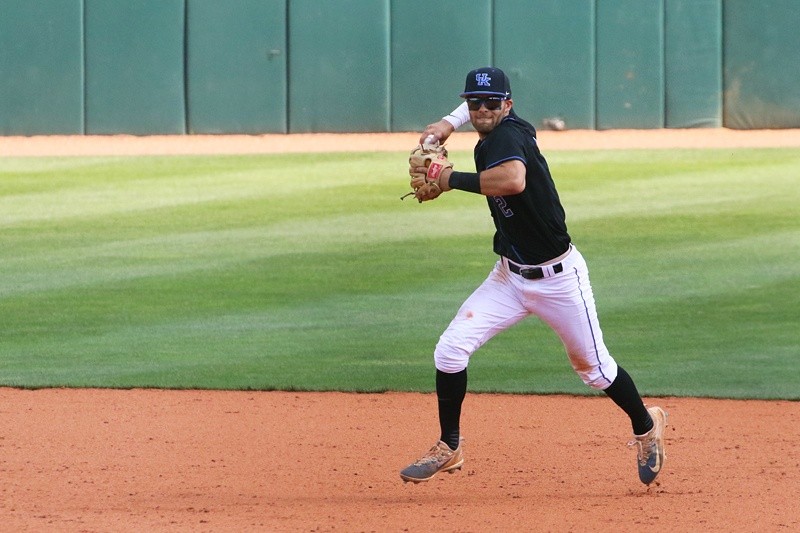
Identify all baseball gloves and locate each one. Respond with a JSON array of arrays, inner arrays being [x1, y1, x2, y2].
[[408, 144, 453, 202]]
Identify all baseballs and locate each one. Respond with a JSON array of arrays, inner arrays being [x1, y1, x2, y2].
[[424, 135, 440, 146]]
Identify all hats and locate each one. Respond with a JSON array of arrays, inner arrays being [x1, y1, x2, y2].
[[460, 66, 512, 98]]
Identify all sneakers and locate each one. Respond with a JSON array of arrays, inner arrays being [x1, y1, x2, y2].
[[401, 440, 465, 486], [633, 406, 665, 486]]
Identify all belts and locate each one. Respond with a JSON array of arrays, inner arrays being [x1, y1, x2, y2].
[[508, 262, 563, 280]]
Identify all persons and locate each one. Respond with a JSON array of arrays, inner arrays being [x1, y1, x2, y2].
[[400, 68, 669, 485]]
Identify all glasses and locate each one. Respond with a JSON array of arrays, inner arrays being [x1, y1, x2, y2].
[[465, 99, 504, 111]]
